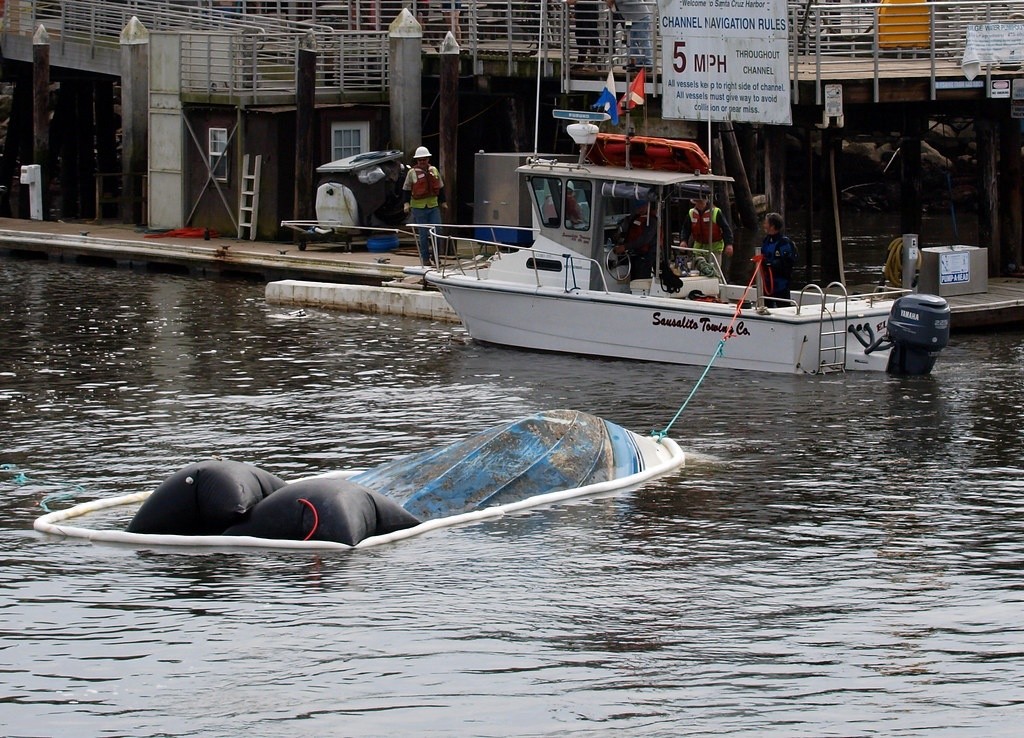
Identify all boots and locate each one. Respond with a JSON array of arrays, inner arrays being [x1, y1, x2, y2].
[[570, 49, 587, 69], [590, 49, 602, 70]]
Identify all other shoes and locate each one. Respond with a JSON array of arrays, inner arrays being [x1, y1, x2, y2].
[[623, 63, 652, 72]]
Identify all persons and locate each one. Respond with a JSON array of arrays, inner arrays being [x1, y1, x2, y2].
[[602, 0, 654, 73], [613, 199, 663, 279], [566, 0, 600, 72], [760, 213, 801, 308], [442, 0, 462, 46], [542, 180, 587, 225], [678, 197, 734, 269], [401, 146, 449, 266]]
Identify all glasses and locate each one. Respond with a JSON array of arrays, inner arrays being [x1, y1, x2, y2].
[[417, 158, 427, 161]]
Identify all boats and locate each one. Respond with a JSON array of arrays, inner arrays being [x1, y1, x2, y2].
[[400, 1, 950, 377]]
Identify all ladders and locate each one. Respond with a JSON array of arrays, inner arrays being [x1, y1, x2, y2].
[[237, 154, 261, 242]]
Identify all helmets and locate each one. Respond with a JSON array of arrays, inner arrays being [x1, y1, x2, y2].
[[413, 146, 432, 158], [558, 180, 575, 190]]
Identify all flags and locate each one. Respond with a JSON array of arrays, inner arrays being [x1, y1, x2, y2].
[[617, 67, 645, 116], [592, 69, 619, 126]]
